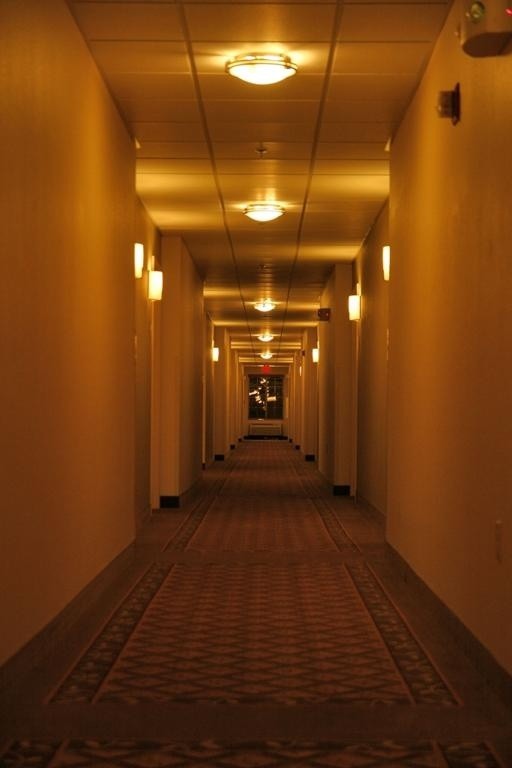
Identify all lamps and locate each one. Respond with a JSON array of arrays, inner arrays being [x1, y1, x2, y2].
[[312, 349, 319, 363], [242, 204, 285, 222], [213, 348, 219, 362], [258, 335, 273, 342], [261, 353, 272, 359], [225, 52, 298, 86], [134, 242, 144, 278], [348, 295, 361, 320], [148, 270, 163, 300], [383, 245, 390, 281], [255, 302, 275, 312]]
